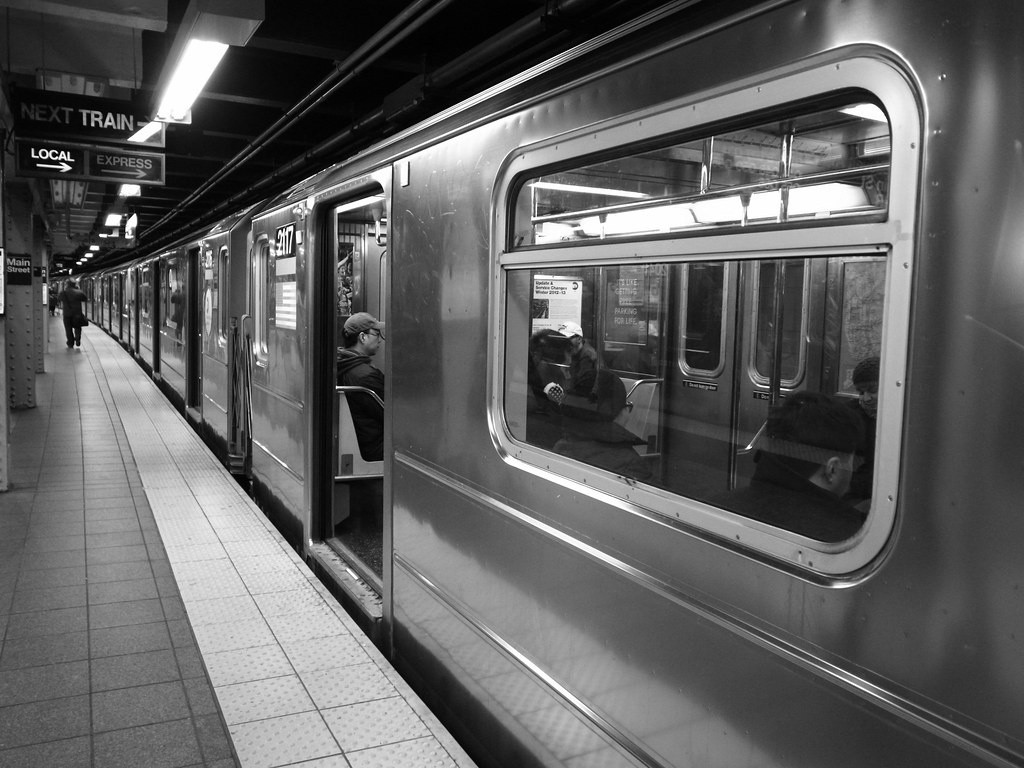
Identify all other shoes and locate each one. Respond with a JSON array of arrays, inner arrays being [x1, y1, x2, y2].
[[68, 345, 73, 348], [76, 339, 80, 345]]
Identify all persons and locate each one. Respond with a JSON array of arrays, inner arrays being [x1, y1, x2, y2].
[[852, 356, 880, 418], [337, 312, 385, 444], [550, 369, 655, 481], [58, 278, 87, 349], [170, 288, 182, 346], [49, 285, 58, 316], [701, 390, 866, 543], [528, 329, 574, 380], [558, 322, 608, 395]]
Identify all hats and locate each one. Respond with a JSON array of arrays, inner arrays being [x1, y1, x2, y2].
[[558, 322, 582, 338], [344, 312, 385, 337]]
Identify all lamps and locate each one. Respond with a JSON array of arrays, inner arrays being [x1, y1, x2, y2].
[[76, 0, 264, 266]]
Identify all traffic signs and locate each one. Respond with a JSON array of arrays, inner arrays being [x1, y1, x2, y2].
[[15, 139, 165, 186]]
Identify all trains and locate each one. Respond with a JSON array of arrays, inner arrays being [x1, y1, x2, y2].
[[48, 0, 1024, 768]]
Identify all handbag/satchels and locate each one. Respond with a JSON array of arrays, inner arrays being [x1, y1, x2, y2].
[[70, 313, 88, 327]]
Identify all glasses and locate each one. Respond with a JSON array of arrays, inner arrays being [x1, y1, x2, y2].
[[366, 332, 379, 337]]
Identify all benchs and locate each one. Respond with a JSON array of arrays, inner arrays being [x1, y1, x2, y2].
[[334, 383, 385, 480], [616, 377, 663, 458]]
